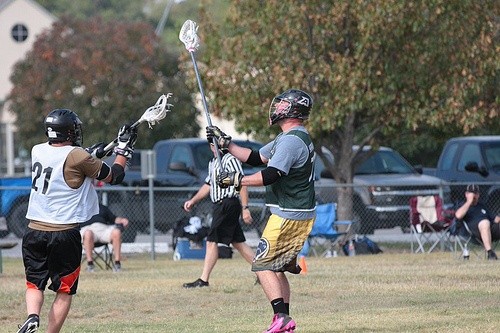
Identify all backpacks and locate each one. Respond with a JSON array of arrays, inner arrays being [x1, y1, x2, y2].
[[343, 235, 383, 255]]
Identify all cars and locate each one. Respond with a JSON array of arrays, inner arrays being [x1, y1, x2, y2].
[[319, 146, 452, 233], [436, 135, 500, 244]]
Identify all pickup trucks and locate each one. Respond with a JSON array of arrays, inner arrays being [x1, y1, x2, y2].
[[2, 138, 343, 244]]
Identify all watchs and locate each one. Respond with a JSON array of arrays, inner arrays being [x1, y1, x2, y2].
[[242, 206, 248, 209]]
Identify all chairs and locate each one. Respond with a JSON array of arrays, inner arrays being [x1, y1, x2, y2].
[[409, 196, 487, 258], [81, 242, 114, 271], [300, 203, 355, 258]]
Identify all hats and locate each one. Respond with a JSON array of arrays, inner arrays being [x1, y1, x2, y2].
[[466, 183, 480, 192]]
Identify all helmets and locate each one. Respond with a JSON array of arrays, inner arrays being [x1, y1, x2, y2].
[[267, 88, 313, 125], [44, 108, 84, 146]]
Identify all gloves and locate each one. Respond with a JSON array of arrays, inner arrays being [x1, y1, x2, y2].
[[85, 142, 113, 160], [205, 125, 232, 155], [215, 170, 243, 194], [113, 124, 138, 160]]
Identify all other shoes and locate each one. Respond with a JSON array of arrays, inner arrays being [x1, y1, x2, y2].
[[114, 264, 122, 273], [183, 278, 209, 289], [488, 251, 497, 260], [16, 315, 40, 333], [86, 265, 95, 272]]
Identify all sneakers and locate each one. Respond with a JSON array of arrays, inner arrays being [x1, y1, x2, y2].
[[265, 313, 296, 333]]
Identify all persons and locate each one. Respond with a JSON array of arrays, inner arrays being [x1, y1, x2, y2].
[[181, 131, 264, 288], [16, 108, 137, 333], [454, 184, 500, 260], [204, 88, 318, 333]]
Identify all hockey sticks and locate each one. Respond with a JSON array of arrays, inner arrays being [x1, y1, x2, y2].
[[104, 93, 171, 151], [178, 19, 224, 174]]
